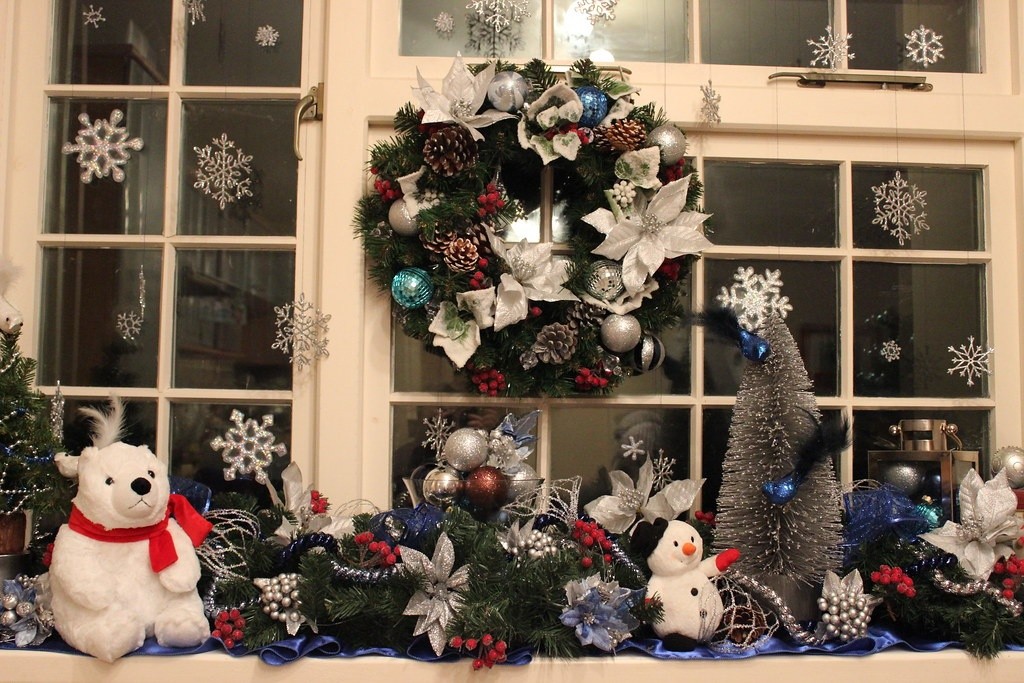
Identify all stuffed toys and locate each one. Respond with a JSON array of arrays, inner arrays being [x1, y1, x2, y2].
[[632, 520, 741, 651], [49, 440, 212, 664]]
